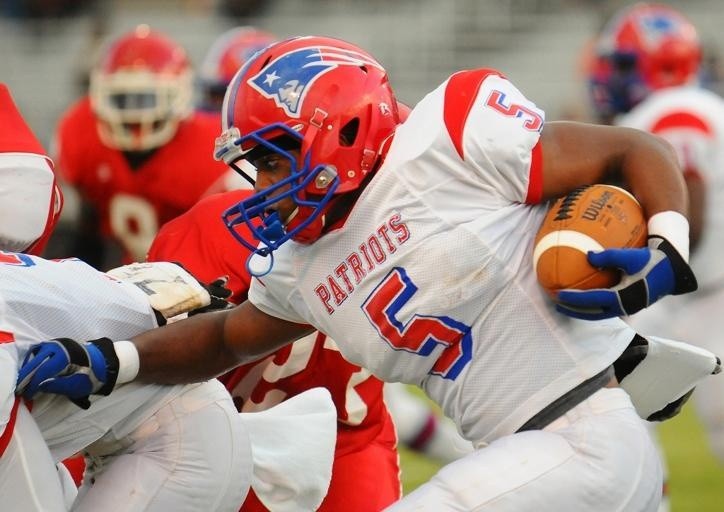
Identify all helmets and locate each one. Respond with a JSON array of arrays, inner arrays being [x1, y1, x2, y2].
[[219, 33, 400, 198], [87, 24, 194, 155], [586, 2, 703, 89]]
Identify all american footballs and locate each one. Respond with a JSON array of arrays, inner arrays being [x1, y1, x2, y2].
[[533, 184, 648, 302]]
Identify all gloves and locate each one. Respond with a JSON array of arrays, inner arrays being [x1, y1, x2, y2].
[[551, 209, 700, 336], [12, 335, 143, 410]]
